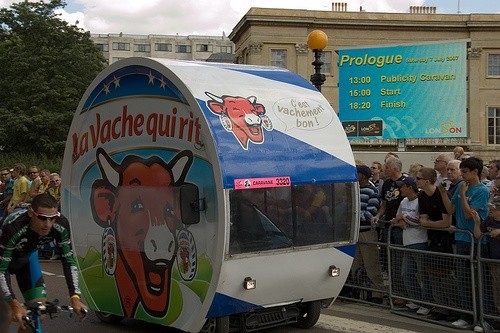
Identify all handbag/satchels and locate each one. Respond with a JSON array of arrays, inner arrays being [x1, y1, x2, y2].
[[423, 237, 453, 268]]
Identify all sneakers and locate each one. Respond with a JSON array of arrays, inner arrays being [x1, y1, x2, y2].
[[474, 321, 493, 332], [451, 319, 475, 330], [406, 302, 435, 315]]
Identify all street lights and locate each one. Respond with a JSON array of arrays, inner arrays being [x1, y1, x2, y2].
[[307, 30, 329, 91]]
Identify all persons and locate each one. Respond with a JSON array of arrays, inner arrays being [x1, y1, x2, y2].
[[0, 193, 88, 333], [471, 197, 500, 333], [247, 183, 352, 225], [0, 166, 61, 260], [391, 177, 431, 315], [417, 167, 455, 322], [352, 166, 384, 303], [438, 157, 490, 332], [355, 146, 500, 206], [370, 156, 412, 306]]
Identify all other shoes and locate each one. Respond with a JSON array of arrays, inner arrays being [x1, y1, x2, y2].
[[37, 254, 61, 260], [490, 328, 500, 333], [341, 295, 410, 305], [427, 312, 459, 322]]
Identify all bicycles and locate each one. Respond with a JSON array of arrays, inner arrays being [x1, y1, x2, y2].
[[14, 203, 55, 258], [11, 300, 86, 333]]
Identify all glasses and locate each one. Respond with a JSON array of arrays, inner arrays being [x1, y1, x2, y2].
[[29, 171, 38, 174], [1, 172, 10, 176], [434, 159, 443, 162], [416, 176, 427, 180], [371, 167, 378, 169], [30, 208, 61, 221], [40, 175, 46, 178], [50, 179, 59, 183]]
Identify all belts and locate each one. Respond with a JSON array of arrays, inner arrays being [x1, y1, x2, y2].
[[360, 227, 374, 232]]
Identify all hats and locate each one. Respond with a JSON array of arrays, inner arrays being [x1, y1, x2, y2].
[[396, 176, 416, 187], [488, 195, 500, 210], [356, 165, 372, 178]]
